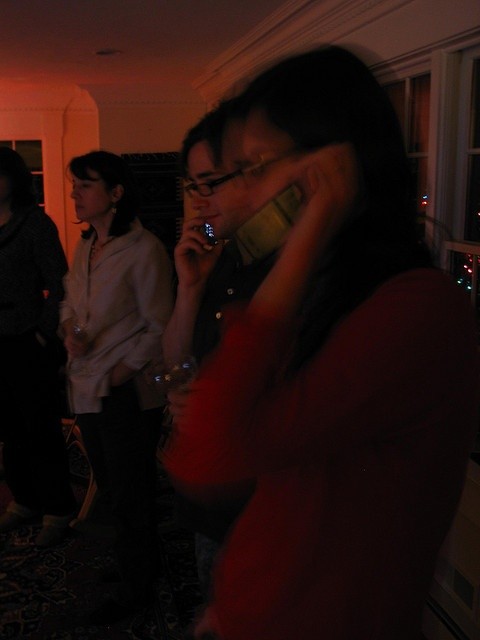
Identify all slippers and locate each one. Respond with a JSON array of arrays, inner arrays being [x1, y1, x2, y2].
[[35, 514, 70, 549], [0, 500, 31, 531]]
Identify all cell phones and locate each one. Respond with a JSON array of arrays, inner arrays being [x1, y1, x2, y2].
[[192, 222, 220, 251], [234, 182, 310, 262]]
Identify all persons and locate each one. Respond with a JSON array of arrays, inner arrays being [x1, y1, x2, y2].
[[57, 151, 173, 622], [0, 144, 73, 547], [160, 45, 478, 640], [161, 89, 284, 381]]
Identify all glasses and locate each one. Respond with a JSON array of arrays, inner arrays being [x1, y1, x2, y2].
[[185, 168, 242, 198], [237, 149, 293, 181]]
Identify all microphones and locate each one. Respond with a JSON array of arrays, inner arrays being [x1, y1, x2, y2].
[[67, 317, 89, 366], [159, 356, 199, 435]]
